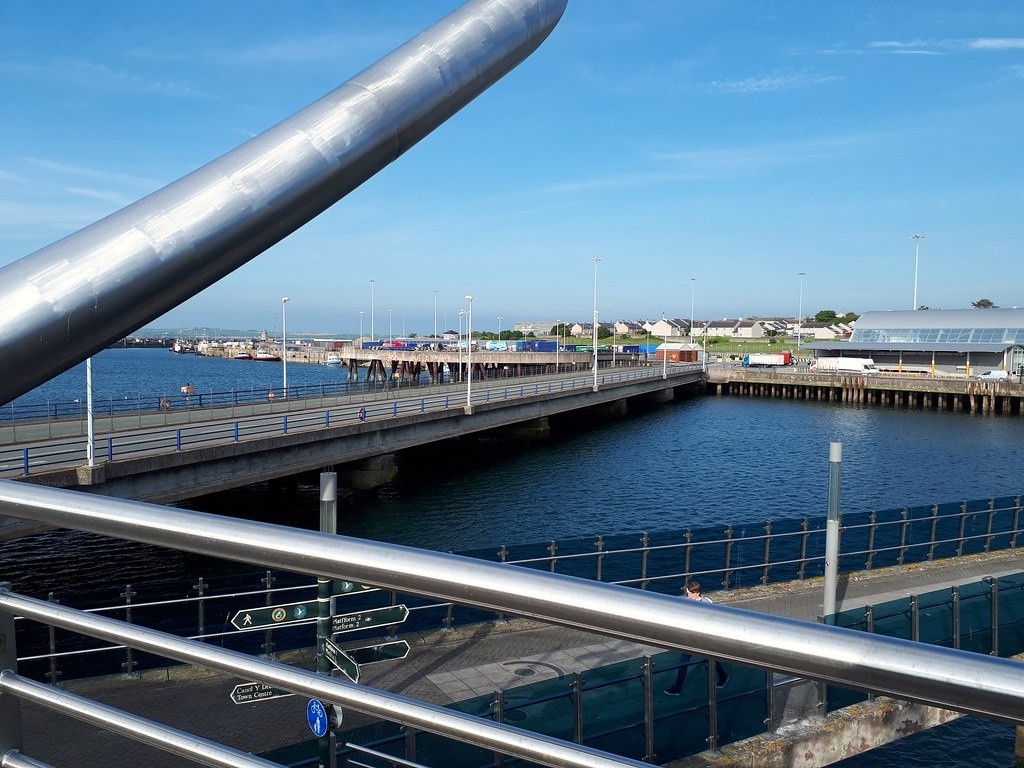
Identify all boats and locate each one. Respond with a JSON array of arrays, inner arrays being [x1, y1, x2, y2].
[[233, 352, 253, 359], [322, 352, 343, 368], [253, 349, 281, 361]]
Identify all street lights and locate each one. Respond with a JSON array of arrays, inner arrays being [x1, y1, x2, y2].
[[703, 323, 706, 368], [497, 316, 504, 341], [589, 257, 601, 392], [360, 278, 448, 352], [465, 296, 473, 407], [797, 272, 806, 350], [690, 278, 695, 344], [663, 319, 668, 375], [281, 297, 290, 399], [458, 309, 464, 382], [910, 233, 926, 310], [612, 324, 617, 368], [556, 320, 560, 373]]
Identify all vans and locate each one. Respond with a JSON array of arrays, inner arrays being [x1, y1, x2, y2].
[[977, 370, 1008, 382]]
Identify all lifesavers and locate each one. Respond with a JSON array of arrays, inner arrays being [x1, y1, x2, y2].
[[161, 399, 171, 409]]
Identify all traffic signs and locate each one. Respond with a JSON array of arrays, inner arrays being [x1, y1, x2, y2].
[[231, 600, 319, 630], [229, 682, 295, 704], [325, 638, 361, 684], [333, 640, 410, 669], [332, 604, 409, 634]]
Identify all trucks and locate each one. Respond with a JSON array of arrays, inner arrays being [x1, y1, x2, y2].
[[809, 357, 881, 377]]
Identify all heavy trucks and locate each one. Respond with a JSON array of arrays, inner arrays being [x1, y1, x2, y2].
[[742, 353, 791, 368]]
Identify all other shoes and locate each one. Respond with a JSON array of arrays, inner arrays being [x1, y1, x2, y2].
[[664, 688, 681, 695], [716, 675, 729, 689]]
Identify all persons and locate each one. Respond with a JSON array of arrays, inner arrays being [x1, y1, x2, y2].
[[662, 580, 731, 696]]
